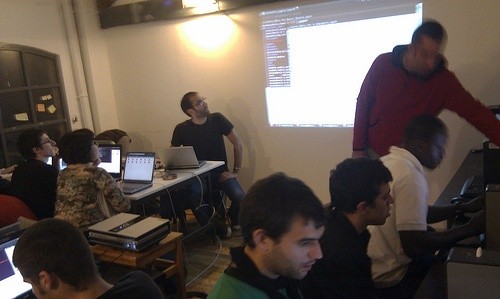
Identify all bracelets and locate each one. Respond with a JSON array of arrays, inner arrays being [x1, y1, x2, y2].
[[234, 167, 241, 170]]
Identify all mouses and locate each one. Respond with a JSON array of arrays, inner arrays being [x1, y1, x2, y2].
[[450, 196, 470, 202]]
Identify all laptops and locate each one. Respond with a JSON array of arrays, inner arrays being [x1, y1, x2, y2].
[[0, 229, 37, 299], [120, 151, 156, 193], [159, 146, 206, 168], [98, 144, 122, 178]]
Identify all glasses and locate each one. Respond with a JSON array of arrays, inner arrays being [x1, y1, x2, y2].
[[191, 97, 207, 110], [36, 138, 51, 148]]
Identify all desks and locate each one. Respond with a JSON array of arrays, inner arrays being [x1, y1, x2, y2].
[[385, 148, 500, 299], [94, 157, 225, 246], [89, 231, 185, 299]]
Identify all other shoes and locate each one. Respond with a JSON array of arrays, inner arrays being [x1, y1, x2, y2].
[[228, 209, 242, 230], [220, 223, 232, 238]]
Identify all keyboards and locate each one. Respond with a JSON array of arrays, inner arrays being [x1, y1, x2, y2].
[[460, 175, 485, 198]]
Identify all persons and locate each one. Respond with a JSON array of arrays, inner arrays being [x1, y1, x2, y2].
[[207, 172, 334, 299], [12, 219, 166, 299], [170, 91, 245, 238], [10, 127, 60, 220], [53, 129, 132, 228], [352, 20, 500, 161], [368, 114, 485, 299], [293, 158, 413, 299]]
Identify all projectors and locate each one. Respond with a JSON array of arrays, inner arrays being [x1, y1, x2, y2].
[[86, 212, 170, 251]]
